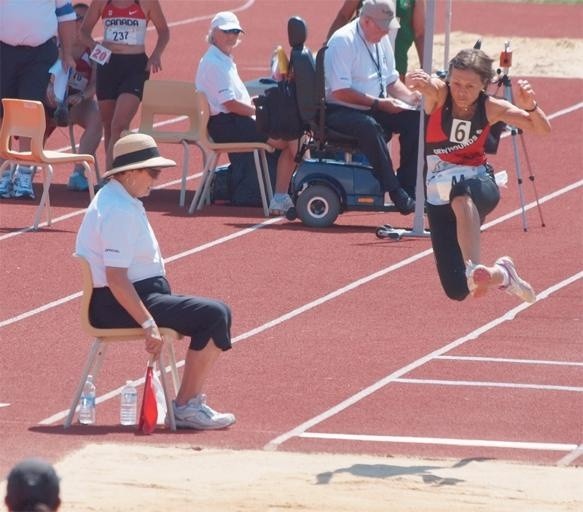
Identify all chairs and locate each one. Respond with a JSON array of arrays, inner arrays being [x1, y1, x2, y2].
[[187, 89, 276, 216], [287, 15, 358, 144], [119, 79, 212, 207], [62, 252, 179, 433], [1, 97, 96, 227]]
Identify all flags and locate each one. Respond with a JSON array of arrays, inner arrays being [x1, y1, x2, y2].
[[134, 351, 161, 439]]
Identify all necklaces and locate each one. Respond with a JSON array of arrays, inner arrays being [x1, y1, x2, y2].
[[461, 103, 474, 112]]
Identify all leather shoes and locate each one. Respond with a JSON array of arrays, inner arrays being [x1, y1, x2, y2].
[[391, 186, 415, 214]]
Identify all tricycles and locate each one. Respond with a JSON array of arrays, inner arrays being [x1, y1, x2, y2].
[[286, 16, 483, 224]]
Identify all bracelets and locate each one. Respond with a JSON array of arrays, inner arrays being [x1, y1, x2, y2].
[[141, 318, 156, 331], [523, 102, 540, 113]]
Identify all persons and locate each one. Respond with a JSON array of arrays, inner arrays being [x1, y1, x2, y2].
[[0, 0, 170, 199], [322, 0, 426, 215], [74, 132, 239, 432], [196, 11, 306, 215], [2, 457, 64, 511], [404, 44, 555, 303]]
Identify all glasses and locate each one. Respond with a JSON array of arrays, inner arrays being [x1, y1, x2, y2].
[[223, 29, 240, 33], [144, 167, 161, 179]]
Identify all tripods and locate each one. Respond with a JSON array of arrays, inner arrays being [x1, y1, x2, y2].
[[491, 74, 545, 232]]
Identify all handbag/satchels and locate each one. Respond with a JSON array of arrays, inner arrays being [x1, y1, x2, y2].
[[252, 84, 304, 141]]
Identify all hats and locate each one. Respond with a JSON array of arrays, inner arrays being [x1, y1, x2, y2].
[[361, 0, 401, 30], [101, 134, 176, 179], [212, 12, 245, 33]]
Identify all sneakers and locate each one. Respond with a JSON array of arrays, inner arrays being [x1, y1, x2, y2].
[[466, 266, 491, 297], [0, 172, 90, 198], [495, 256, 536, 304], [270, 196, 295, 216], [165, 395, 235, 430]]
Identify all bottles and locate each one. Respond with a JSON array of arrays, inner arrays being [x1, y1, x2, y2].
[[270, 48, 283, 83], [119, 381, 137, 425], [78, 374, 95, 423]]
